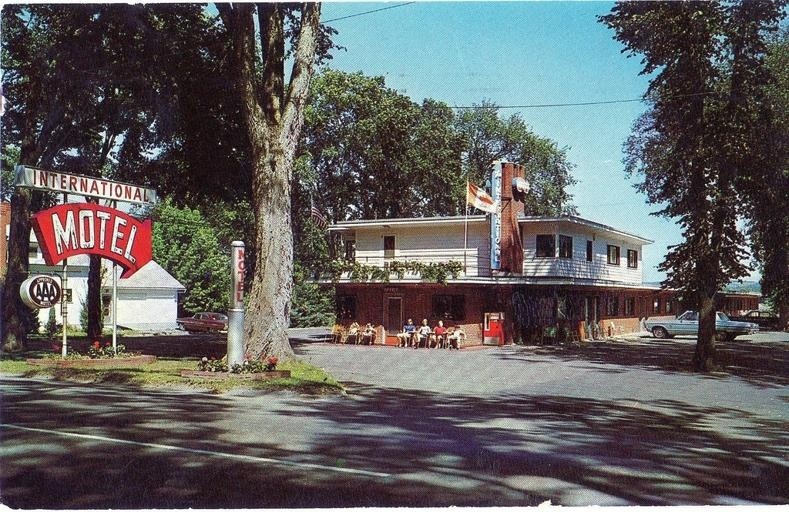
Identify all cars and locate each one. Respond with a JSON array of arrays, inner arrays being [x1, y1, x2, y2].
[[729, 307, 780, 329], [644, 310, 761, 343], [176, 312, 228, 333]]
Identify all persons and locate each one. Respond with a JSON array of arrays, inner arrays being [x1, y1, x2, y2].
[[431, 320, 447, 349], [397, 318, 415, 347], [447, 325, 467, 350], [412, 318, 431, 349], [363, 321, 376, 345], [349, 320, 360, 343]]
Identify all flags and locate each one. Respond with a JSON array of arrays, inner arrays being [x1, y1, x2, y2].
[[470, 182, 496, 214]]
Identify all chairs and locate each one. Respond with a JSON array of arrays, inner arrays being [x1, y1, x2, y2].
[[324, 328, 377, 345], [399, 326, 462, 350]]
